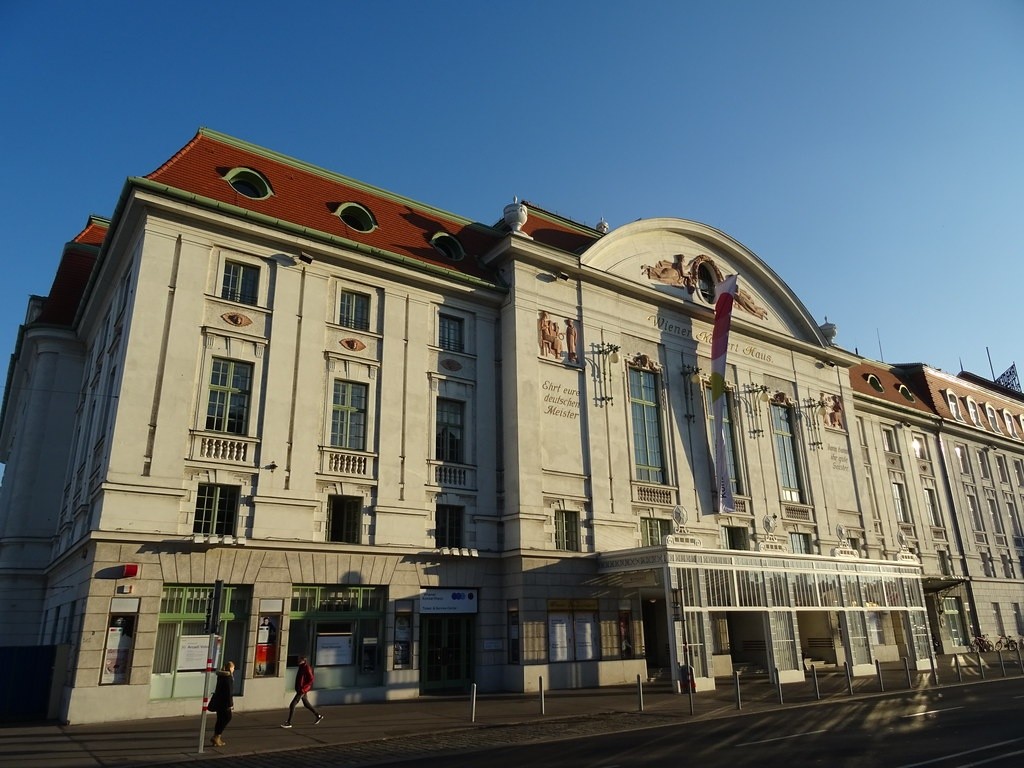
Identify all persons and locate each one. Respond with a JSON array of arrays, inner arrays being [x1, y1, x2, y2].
[[818, 392, 845, 429], [675, 255, 695, 294], [210, 661, 234, 746], [260, 617, 277, 642], [281, 656, 325, 728], [539, 311, 578, 362]]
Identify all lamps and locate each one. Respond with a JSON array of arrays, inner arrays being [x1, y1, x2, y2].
[[681, 364, 702, 424], [749, 382, 771, 437], [808, 397, 828, 451], [599, 343, 621, 406]]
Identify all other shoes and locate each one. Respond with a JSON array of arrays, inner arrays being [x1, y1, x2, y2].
[[280, 720, 293, 729], [314, 713, 324, 725], [210, 735, 227, 747]]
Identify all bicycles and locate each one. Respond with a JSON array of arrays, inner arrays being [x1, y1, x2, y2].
[[996, 635, 1017, 652], [968, 634, 994, 654]]
